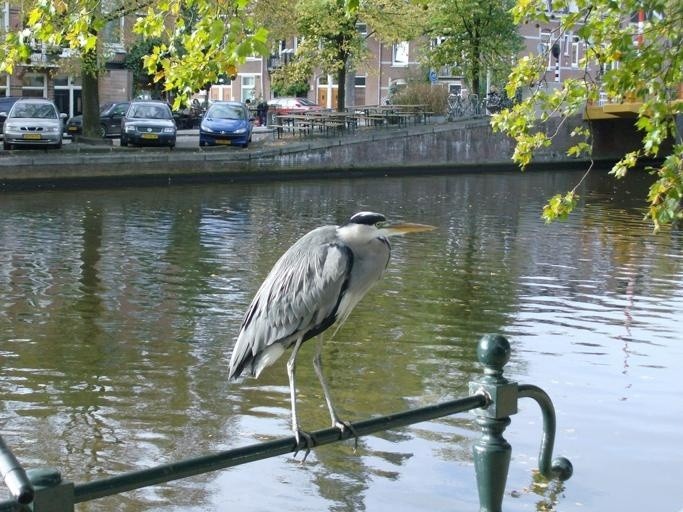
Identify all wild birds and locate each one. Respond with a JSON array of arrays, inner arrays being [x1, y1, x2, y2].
[[227, 211, 440, 464]]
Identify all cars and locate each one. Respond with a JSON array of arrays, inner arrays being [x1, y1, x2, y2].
[[64, 93, 129, 149], [266, 96, 324, 124], [120, 101, 177, 146], [198, 98, 252, 148], [1, 95, 68, 149]]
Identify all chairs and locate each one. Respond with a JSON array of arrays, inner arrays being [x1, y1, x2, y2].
[[443, 92, 503, 120], [269, 105, 435, 140]]
